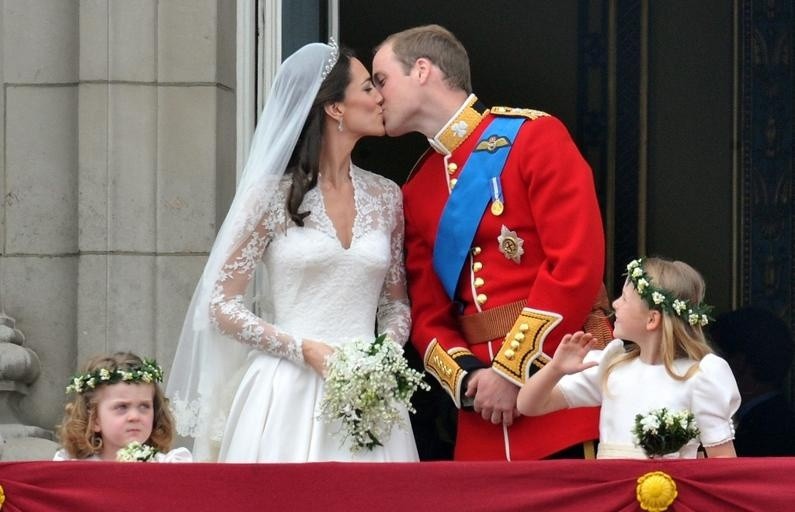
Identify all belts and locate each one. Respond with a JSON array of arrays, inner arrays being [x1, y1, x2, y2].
[[461, 278, 611, 350]]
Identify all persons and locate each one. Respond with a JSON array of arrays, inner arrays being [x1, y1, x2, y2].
[[516, 257, 741, 459], [164, 35, 421, 463], [51, 351, 194, 463], [369, 22, 619, 462]]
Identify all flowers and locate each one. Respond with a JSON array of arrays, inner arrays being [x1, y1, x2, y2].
[[314, 335, 436, 454], [64, 357, 163, 398], [621, 255, 710, 327], [632, 407, 699, 457], [115, 441, 160, 464]]
